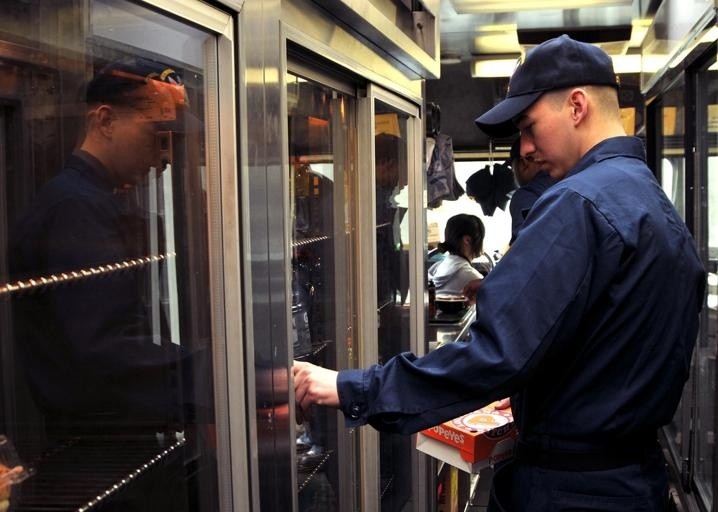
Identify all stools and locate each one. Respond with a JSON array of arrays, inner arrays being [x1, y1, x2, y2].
[[514, 434, 657, 473]]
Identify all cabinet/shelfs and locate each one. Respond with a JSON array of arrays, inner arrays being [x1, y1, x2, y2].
[[427, 280, 436, 317]]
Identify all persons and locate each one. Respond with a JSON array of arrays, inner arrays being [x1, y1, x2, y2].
[[375, 131, 407, 366], [428, 212, 485, 294], [12, 69, 190, 511], [294, 32, 707, 511], [463, 134, 558, 305]]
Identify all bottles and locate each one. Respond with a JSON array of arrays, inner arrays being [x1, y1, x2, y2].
[[476, 35, 614, 136], [500, 137, 520, 168], [86, 58, 204, 138]]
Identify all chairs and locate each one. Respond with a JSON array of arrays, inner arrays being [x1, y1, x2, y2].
[[296, 433, 337, 473]]
